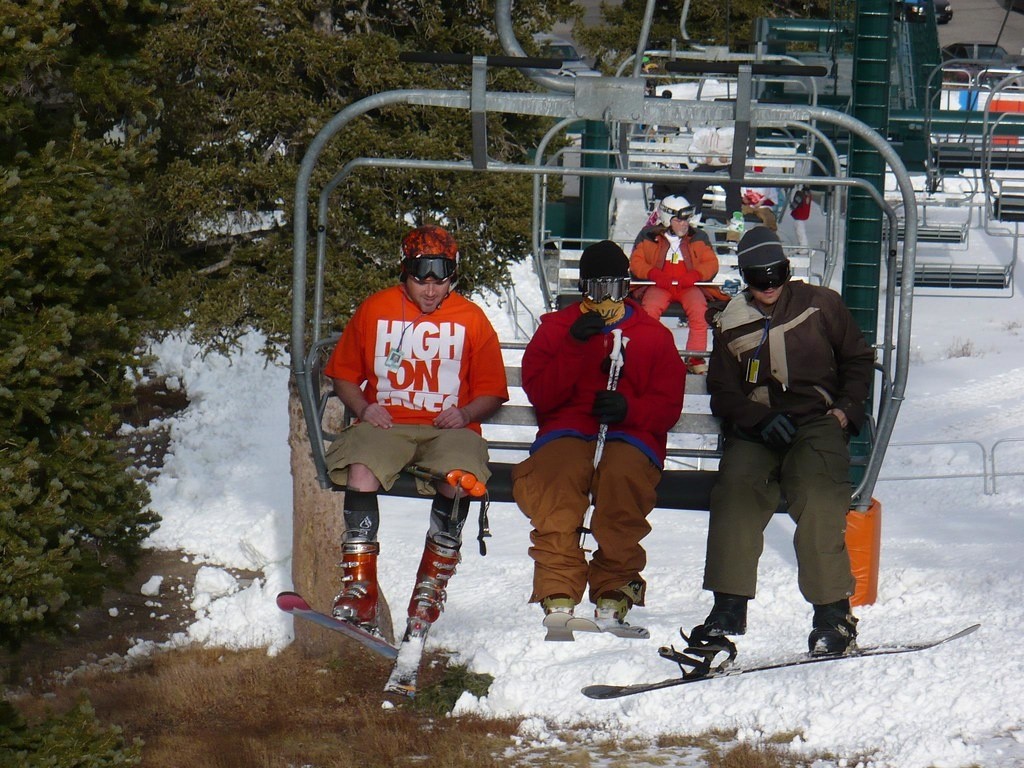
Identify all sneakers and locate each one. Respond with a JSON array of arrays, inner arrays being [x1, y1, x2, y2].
[[597, 580, 644, 620], [541, 593, 574, 615], [809, 606, 859, 655], [684, 356, 708, 375], [692, 597, 747, 636]]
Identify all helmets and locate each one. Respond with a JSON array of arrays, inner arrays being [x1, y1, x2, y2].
[[657, 195, 696, 228], [401, 225, 457, 259]]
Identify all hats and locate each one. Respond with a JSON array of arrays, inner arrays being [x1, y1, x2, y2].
[[579, 240, 630, 279], [737, 226, 786, 267]]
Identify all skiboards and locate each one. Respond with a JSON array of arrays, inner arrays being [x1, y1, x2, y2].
[[273, 589, 432, 699], [542, 611, 651, 642]]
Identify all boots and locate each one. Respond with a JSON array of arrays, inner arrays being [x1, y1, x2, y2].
[[407, 530, 462, 623], [332, 538, 380, 626]]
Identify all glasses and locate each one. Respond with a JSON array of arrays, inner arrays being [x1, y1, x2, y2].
[[739, 259, 791, 292], [403, 254, 457, 285], [578, 277, 631, 304]]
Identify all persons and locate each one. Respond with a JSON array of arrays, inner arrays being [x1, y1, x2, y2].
[[325, 224, 511, 624], [516, 243, 688, 621], [552, 48, 566, 58], [791, 183, 812, 254], [687, 125, 750, 219], [633, 62, 680, 142], [630, 196, 720, 375], [685, 228, 873, 657]]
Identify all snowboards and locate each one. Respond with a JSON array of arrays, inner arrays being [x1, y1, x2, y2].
[[580, 620, 982, 702]]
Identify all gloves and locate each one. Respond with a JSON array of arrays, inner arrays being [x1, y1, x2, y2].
[[569, 311, 604, 343], [677, 270, 703, 289], [760, 414, 795, 450], [648, 267, 672, 289], [592, 390, 627, 424]]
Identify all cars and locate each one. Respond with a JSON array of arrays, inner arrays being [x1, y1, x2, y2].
[[532, 32, 603, 78]]
